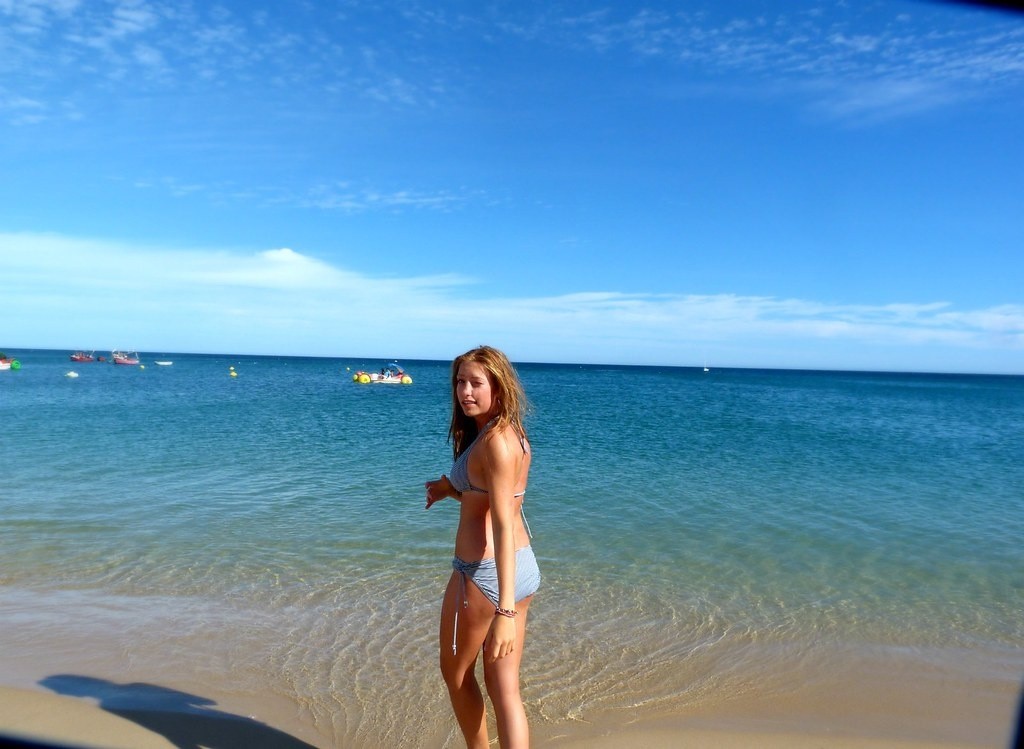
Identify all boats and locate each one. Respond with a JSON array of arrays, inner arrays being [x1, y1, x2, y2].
[[97, 356, 105, 361], [0, 353, 21, 370], [112, 352, 140, 365], [353, 363, 413, 385], [69, 352, 94, 362], [154, 361, 173, 365]]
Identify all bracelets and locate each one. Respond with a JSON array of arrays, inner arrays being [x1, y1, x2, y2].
[[494, 606, 518, 618]]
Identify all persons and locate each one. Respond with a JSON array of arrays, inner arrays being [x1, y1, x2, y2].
[[424, 345, 541, 749], [381, 367, 394, 379]]
